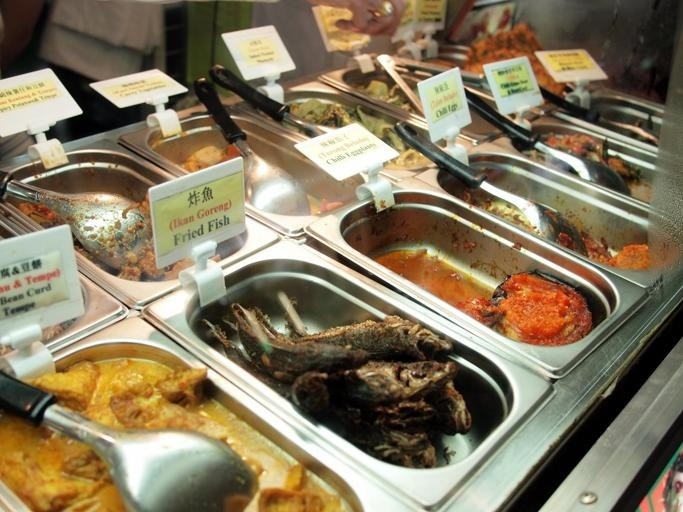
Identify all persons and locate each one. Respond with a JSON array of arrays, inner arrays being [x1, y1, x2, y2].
[[35, 0, 410, 145]]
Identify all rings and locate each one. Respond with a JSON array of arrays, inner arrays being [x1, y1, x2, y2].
[[374, 1, 394, 16]]
